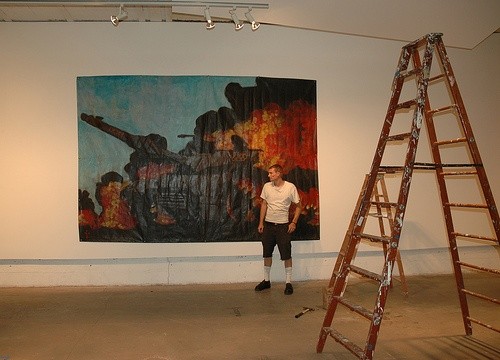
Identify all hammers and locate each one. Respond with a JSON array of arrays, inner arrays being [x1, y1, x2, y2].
[[295, 307, 315, 318]]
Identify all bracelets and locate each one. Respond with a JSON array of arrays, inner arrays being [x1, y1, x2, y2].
[[292, 222, 296, 225]]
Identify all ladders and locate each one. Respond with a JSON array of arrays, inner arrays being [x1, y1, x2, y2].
[[329, 174, 417, 298], [319, 33, 500, 360]]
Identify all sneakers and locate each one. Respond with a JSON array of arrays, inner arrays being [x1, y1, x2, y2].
[[284, 283, 293, 296], [255, 279, 270, 291]]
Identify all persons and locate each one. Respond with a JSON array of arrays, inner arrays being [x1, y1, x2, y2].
[[254, 164, 304, 295]]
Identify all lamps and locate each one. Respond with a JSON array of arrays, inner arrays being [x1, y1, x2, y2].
[[228, 6, 244, 30], [110, 3, 128, 26], [203, 6, 216, 30], [245, 7, 261, 30]]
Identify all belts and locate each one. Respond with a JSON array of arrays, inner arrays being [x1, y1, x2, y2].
[[265, 221, 283, 226]]
